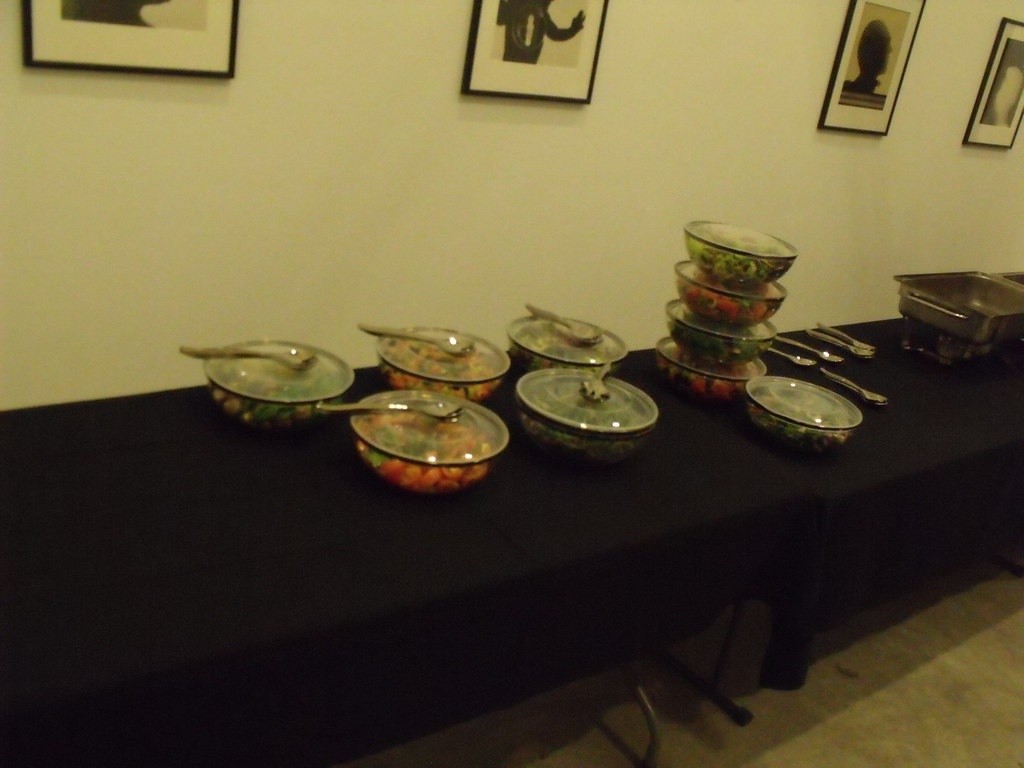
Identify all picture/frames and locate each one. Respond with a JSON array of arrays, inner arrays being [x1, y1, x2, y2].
[[816, 1, 928, 138], [460, 1, 609, 105], [22, 0, 238, 79], [962, 17, 1024, 150]]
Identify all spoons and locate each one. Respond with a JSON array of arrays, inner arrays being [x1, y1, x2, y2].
[[584, 362, 614, 402], [359, 322, 473, 354], [806, 329, 873, 355], [819, 366, 889, 405], [183, 344, 313, 370], [319, 402, 463, 421], [527, 302, 600, 347], [817, 322, 877, 357], [775, 336, 845, 362], [766, 344, 817, 368]]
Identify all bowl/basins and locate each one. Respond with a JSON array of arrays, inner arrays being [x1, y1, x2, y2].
[[376, 328, 510, 402], [204, 341, 354, 425], [512, 317, 628, 371], [674, 262, 786, 326], [352, 389, 510, 492], [517, 373, 659, 456], [746, 377, 863, 451], [665, 301, 779, 366], [657, 340, 767, 400], [687, 220, 799, 286]]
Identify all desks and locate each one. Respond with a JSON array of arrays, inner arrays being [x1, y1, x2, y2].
[[598, 314, 1024, 727], [1, 316, 757, 768]]
